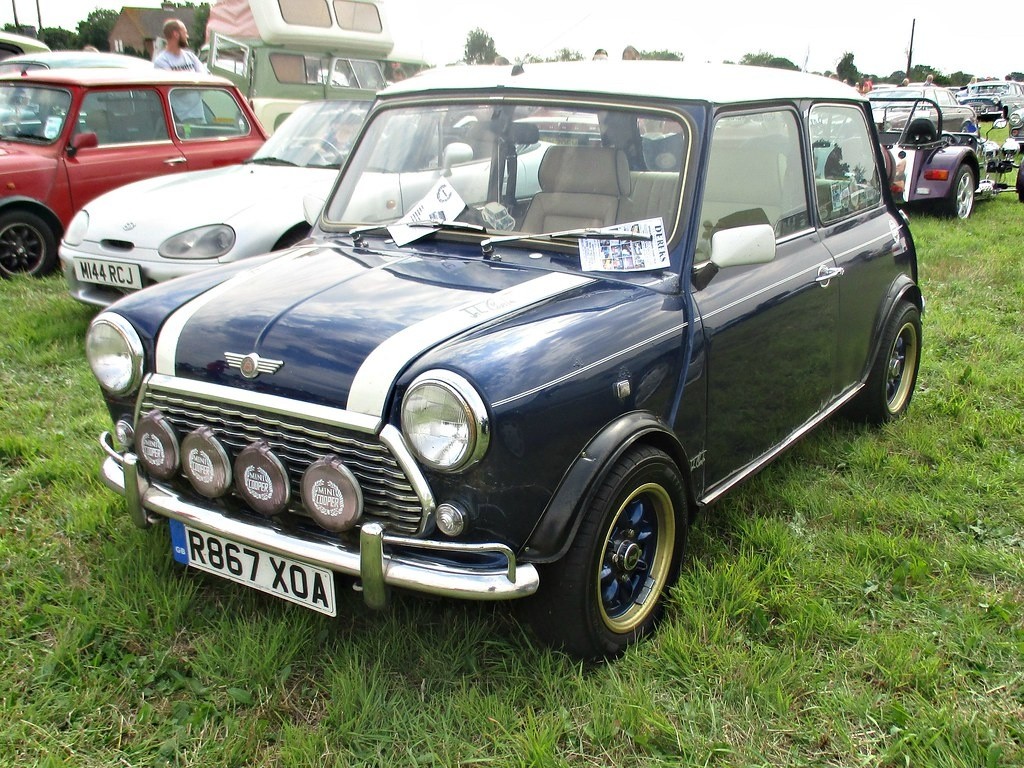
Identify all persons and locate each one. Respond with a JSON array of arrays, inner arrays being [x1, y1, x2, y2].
[[829, 74, 873, 94], [969, 77, 977, 85], [592, 48, 608, 60], [622, 46, 642, 60], [985, 77, 993, 89], [889, 150, 907, 203], [923, 74, 933, 87], [1005, 75, 1013, 81], [495, 56, 510, 65], [897, 77, 909, 87], [153, 17, 212, 126], [392, 68, 408, 81]]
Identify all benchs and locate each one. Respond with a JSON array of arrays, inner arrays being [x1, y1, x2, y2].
[[629, 169, 877, 240]]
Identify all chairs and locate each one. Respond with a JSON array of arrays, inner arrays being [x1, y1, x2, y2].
[[692, 137, 786, 265], [518, 147, 636, 234], [85, 110, 123, 143]]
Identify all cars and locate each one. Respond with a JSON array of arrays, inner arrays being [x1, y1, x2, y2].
[[84, 64, 925, 661], [0, 0, 1024, 308]]
